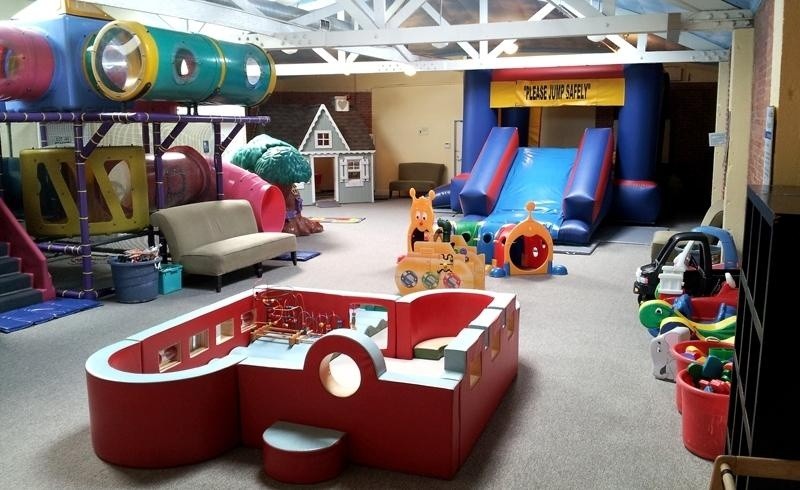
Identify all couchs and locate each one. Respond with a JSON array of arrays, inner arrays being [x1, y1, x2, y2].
[[388, 162, 444, 200], [150, 198, 297, 293]]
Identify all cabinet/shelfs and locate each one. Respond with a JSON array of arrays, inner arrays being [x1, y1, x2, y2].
[[724, 185, 799, 489]]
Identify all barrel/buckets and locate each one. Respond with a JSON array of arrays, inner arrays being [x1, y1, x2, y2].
[[675, 369, 732, 463], [107, 256, 162, 304], [669, 336, 735, 414]]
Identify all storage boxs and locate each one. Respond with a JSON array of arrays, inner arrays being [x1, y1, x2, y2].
[[158, 264, 184, 295]]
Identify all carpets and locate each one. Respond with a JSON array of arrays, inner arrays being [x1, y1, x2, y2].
[[550, 224, 670, 255], [271, 250, 320, 262], [306, 216, 366, 224], [0, 296, 103, 334]]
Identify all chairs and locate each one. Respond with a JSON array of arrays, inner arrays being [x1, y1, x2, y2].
[[650, 200, 723, 266]]
